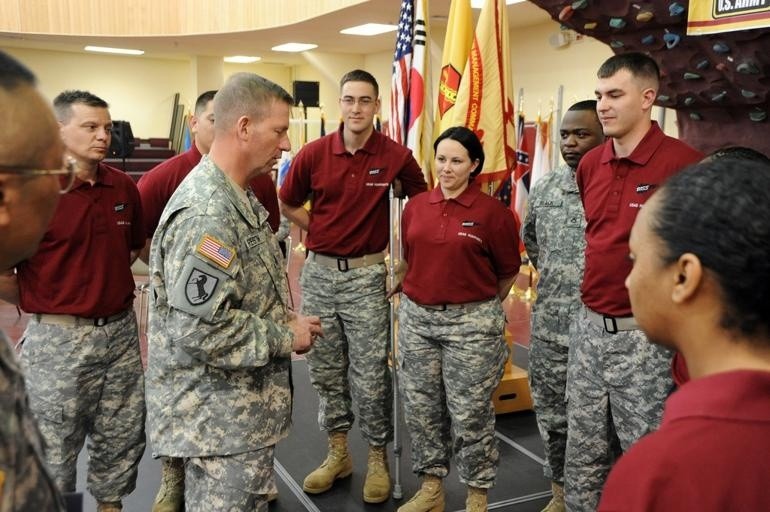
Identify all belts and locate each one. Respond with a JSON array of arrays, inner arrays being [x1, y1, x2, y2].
[[586, 309, 639, 333], [429, 303, 471, 311], [40, 311, 125, 326], [308, 250, 384, 271]]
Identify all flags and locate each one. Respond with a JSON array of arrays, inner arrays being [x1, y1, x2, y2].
[[446, 0, 517, 190], [405, 0, 431, 174], [438, 0, 471, 178], [515, 95, 560, 247], [388, 1, 411, 153]]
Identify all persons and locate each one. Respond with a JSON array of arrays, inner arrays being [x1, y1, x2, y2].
[[275, 67, 422, 502], [1, 56, 80, 511], [521, 100, 603, 511], [151, 70, 303, 512], [562, 52, 709, 512], [392, 127, 517, 512], [7, 87, 153, 511], [134, 90, 282, 512], [599, 143, 770, 512]]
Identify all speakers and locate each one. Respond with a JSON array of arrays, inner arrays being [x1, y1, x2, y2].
[[293, 82, 320, 108], [105, 121, 139, 158]]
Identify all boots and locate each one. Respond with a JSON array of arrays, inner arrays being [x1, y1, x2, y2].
[[541, 483, 566, 511], [303, 432, 353, 494], [466, 485, 487, 511], [397, 475, 445, 512], [153, 459, 184, 512], [363, 445, 391, 503]]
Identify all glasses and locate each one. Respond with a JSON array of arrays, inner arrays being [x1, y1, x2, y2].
[[0, 156, 76, 194], [339, 97, 376, 107]]
